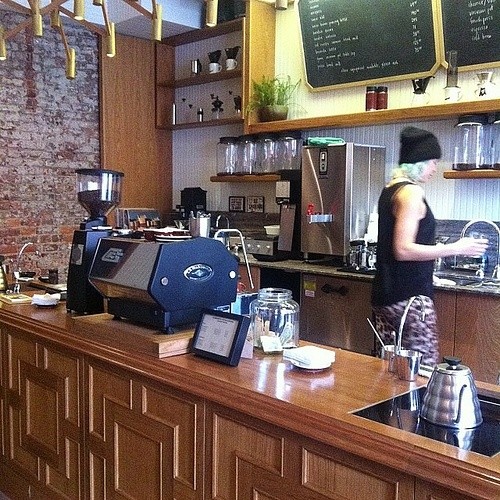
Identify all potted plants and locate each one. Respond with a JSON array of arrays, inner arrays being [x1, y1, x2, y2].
[[245, 72, 308, 122]]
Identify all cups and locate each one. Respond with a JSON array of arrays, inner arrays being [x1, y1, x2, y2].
[[395, 349, 423, 381], [382, 345, 404, 373], [208, 63, 222, 74], [225, 59, 237, 70], [191, 60, 200, 76], [469, 231, 488, 259], [196, 107, 203, 122]]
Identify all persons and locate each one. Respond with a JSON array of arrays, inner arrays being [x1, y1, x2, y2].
[[371, 126, 488, 366]]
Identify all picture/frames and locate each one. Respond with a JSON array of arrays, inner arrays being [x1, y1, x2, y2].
[[246, 195, 264, 213], [228, 195, 245, 212]]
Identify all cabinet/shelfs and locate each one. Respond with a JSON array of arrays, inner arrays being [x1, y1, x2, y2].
[[154, 18, 247, 130], [211, 98, 500, 183], [298, 274, 500, 385]]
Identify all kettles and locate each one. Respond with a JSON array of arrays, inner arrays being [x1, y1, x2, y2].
[[418, 355, 484, 430]]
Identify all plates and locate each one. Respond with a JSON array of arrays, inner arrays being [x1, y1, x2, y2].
[[289, 360, 332, 373], [155, 235, 192, 242]]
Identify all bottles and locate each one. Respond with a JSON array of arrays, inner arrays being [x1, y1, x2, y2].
[[248, 286, 300, 349], [367, 241, 378, 269], [216, 131, 304, 176], [452, 116, 500, 171], [49, 268, 58, 284], [366, 86, 377, 112], [377, 86, 388, 111], [348, 239, 367, 269]]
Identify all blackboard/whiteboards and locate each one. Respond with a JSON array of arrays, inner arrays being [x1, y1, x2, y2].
[[294, 0, 440, 92], [436, 0, 500, 72]]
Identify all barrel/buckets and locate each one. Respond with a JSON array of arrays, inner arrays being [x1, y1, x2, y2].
[[188, 217, 211, 239]]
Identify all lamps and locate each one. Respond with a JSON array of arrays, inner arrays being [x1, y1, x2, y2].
[[0, 0, 217, 79]]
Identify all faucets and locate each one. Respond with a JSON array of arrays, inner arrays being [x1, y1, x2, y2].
[[396, 295, 425, 351], [13, 241, 34, 293], [460, 218, 500, 279]]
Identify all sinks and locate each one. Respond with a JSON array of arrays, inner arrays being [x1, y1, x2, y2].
[[433, 268, 500, 289], [7, 281, 61, 296]]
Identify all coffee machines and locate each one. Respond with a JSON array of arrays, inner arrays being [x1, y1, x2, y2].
[[66, 168, 124, 314]]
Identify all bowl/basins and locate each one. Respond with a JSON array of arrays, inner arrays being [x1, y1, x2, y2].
[[38, 276, 50, 283], [263, 224, 280, 235], [20, 272, 36, 278], [143, 229, 184, 241]]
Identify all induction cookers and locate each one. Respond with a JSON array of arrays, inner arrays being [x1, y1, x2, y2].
[[345, 383, 500, 458]]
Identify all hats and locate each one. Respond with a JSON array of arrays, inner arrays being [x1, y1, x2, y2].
[[397, 125, 442, 164]]
